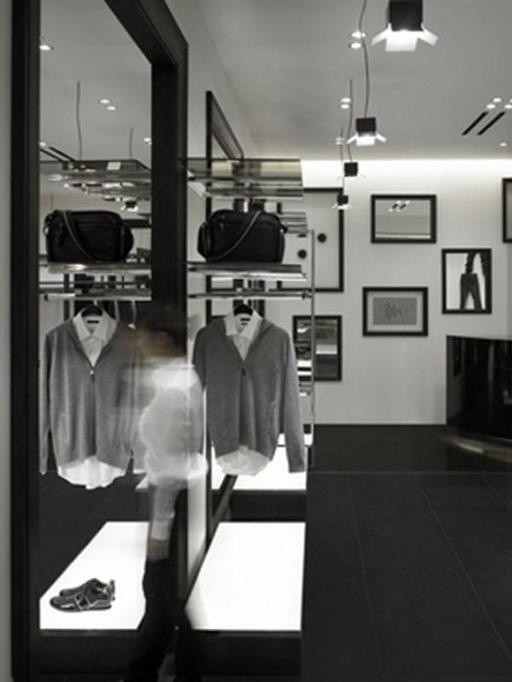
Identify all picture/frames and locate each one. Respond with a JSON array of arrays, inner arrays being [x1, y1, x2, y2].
[[266, 187, 345, 293], [362, 288, 428, 336], [292, 316, 342, 382], [371, 194, 436, 243], [442, 249, 492, 313]]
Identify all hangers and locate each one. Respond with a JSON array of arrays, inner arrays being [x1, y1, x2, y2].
[[75, 300, 114, 328], [231, 298, 257, 323]]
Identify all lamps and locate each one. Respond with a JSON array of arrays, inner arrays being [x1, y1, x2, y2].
[[335, 1, 439, 212]]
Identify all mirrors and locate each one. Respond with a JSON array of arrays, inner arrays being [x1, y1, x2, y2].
[[14, 0, 187, 682], [203, 93, 258, 539]]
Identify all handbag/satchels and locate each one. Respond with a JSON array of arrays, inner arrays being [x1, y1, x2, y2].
[[42, 208, 135, 263], [197, 209, 287, 262]]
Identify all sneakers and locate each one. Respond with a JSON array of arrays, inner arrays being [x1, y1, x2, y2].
[[59, 579, 116, 600], [50, 585, 111, 613]]
